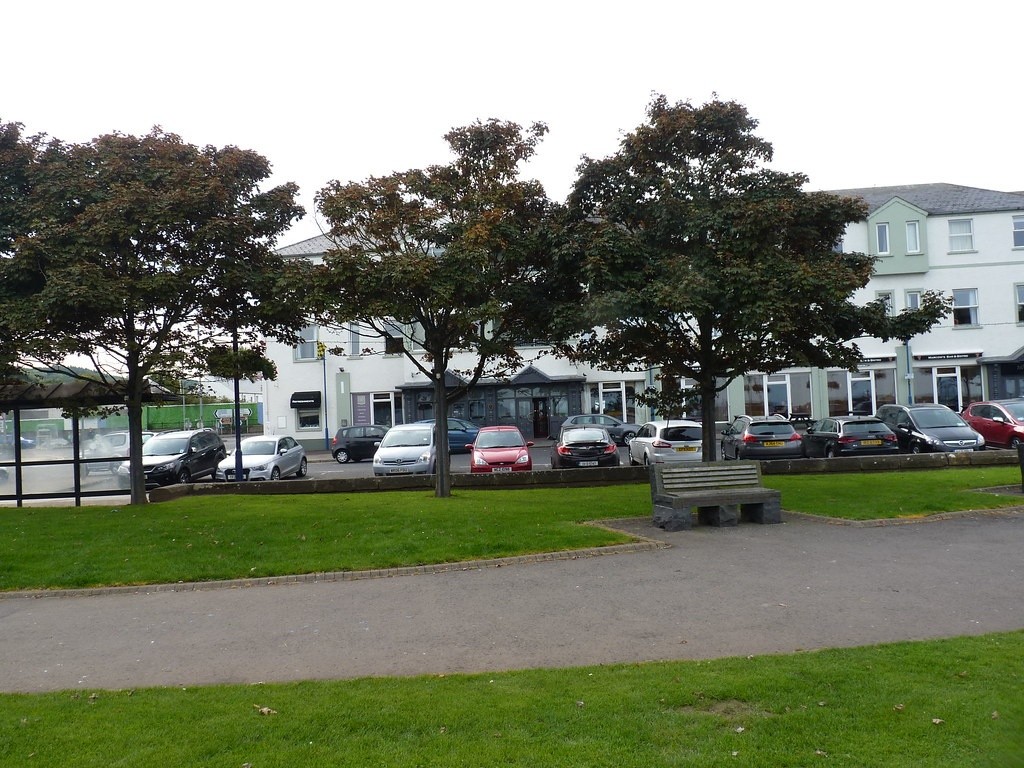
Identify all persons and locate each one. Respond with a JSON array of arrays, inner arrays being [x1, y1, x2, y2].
[[89, 428, 96, 439]]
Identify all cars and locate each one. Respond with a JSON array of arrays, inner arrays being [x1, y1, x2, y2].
[[627, 419, 704, 467], [372, 424, 451, 477], [216, 434, 308, 482], [558, 414, 651, 447], [6, 435, 36, 449], [331, 425, 389, 464], [413, 417, 482, 453], [546, 423, 621, 470], [465, 425, 534, 472], [800, 416, 899, 458]]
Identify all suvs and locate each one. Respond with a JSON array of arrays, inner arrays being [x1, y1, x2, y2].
[[78, 431, 155, 475], [961, 398, 1024, 449], [720, 414, 804, 461], [118, 427, 227, 491], [875, 403, 986, 454]]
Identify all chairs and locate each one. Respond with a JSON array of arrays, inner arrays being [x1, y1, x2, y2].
[[235, 416, 249, 433], [220, 417, 232, 435], [195, 419, 214, 431]]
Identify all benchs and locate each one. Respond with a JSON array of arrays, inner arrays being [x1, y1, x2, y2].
[[649, 460, 781, 532]]
